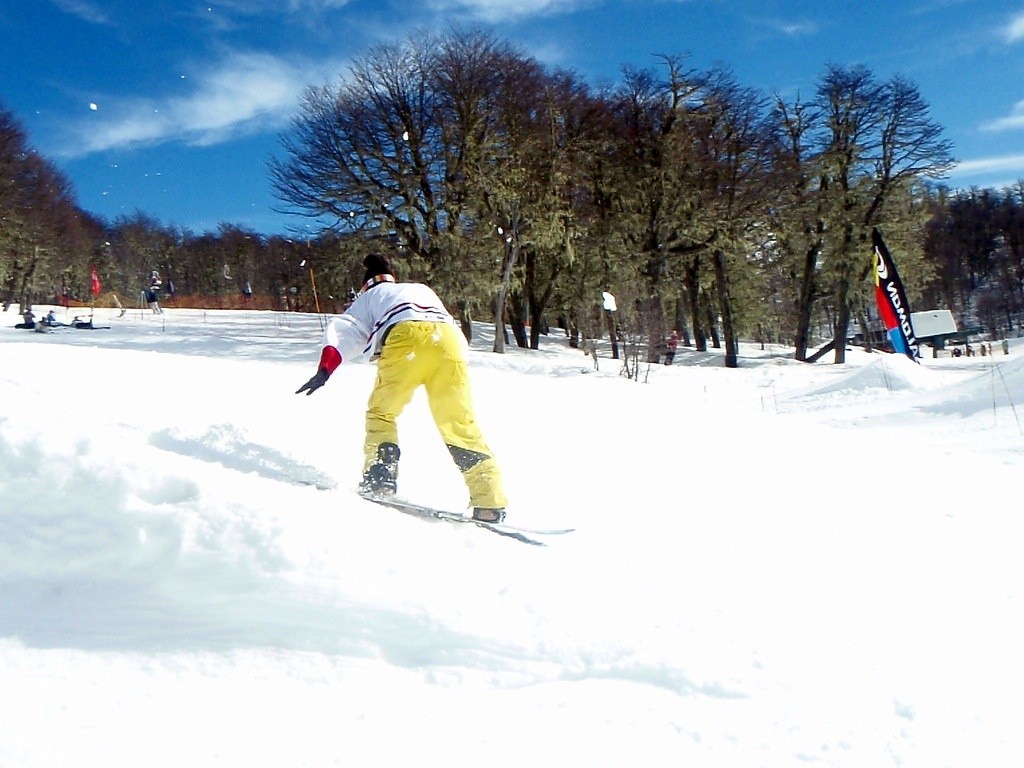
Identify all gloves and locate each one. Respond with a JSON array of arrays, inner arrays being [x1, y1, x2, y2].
[[294, 370, 329, 395]]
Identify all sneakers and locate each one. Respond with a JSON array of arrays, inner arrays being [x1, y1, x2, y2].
[[358, 442, 400, 497], [472, 505, 506, 523]]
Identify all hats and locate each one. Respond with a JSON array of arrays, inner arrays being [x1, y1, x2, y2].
[[362, 253, 395, 291]]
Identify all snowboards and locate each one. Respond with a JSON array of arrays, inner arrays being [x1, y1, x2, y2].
[[358, 485, 577, 539]]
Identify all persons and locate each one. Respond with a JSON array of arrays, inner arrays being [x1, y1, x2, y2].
[[241, 273, 252, 302], [23, 308, 92, 333], [664, 334, 678, 365], [295, 254, 504, 524], [952, 340, 1009, 357], [148, 271, 162, 314]]
